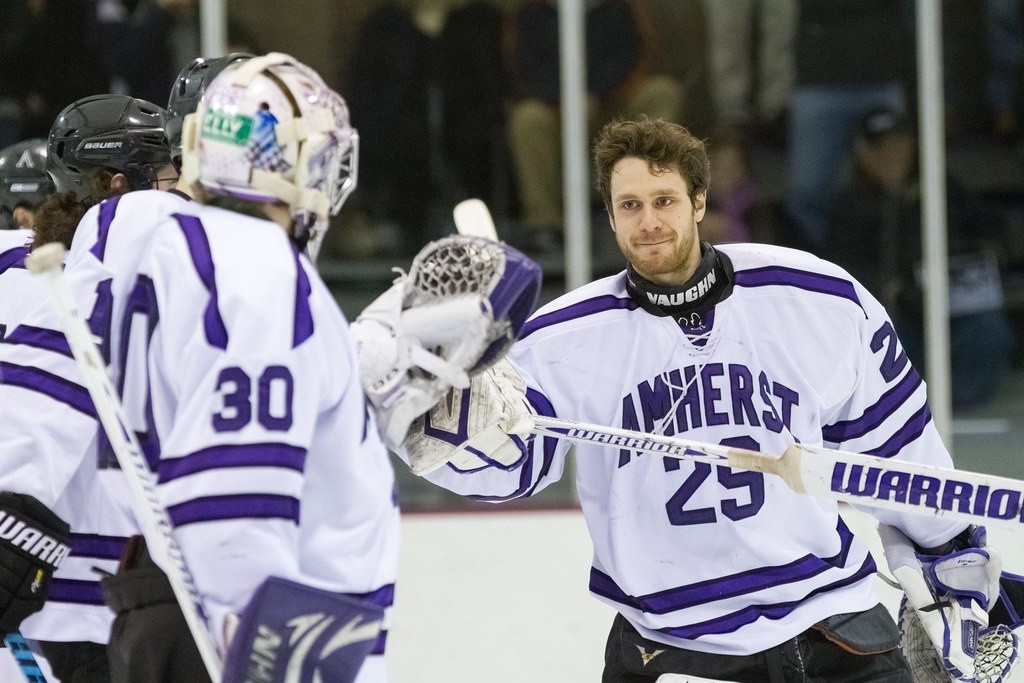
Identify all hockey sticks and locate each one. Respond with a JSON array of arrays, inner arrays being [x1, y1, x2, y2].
[[530, 411, 1024, 529], [25, 241, 224, 683]]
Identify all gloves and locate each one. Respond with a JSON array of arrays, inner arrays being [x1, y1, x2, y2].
[[913, 524, 1003, 681]]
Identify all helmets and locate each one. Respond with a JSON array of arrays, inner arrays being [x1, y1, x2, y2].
[[163, 51, 359, 263], [0, 94, 167, 216]]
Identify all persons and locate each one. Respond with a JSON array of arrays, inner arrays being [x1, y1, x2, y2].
[[0, 52, 539, 683], [349, 115, 1001, 683]]
